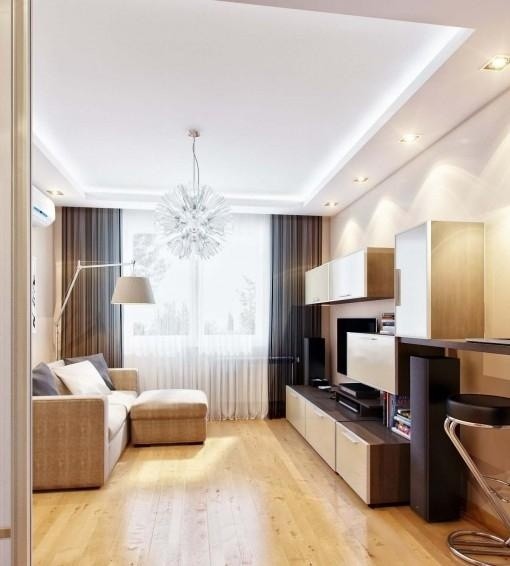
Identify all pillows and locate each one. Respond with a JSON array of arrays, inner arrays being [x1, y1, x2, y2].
[[32, 353, 117, 399]]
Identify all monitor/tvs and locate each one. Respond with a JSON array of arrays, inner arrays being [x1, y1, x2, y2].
[[336, 317, 378, 398]]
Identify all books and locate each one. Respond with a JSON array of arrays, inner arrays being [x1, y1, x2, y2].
[[379, 310, 396, 336], [381, 390, 412, 441]]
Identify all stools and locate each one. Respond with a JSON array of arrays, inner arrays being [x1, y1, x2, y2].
[[441, 394, 510, 565], [130, 387, 209, 447]]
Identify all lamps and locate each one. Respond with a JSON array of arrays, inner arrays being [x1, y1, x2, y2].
[[154, 130, 236, 261], [52, 256, 156, 324]]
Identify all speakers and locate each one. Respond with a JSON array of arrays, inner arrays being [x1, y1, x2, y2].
[[303, 336, 326, 386], [409, 355, 462, 522]]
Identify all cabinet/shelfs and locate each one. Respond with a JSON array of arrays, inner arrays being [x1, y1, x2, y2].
[[284, 384, 410, 506], [394, 219, 486, 339], [303, 248, 396, 306]]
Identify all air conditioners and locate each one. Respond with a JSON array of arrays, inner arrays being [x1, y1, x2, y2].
[[30, 184, 56, 227]]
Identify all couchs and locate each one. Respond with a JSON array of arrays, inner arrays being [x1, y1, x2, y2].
[[29, 366, 139, 491]]
